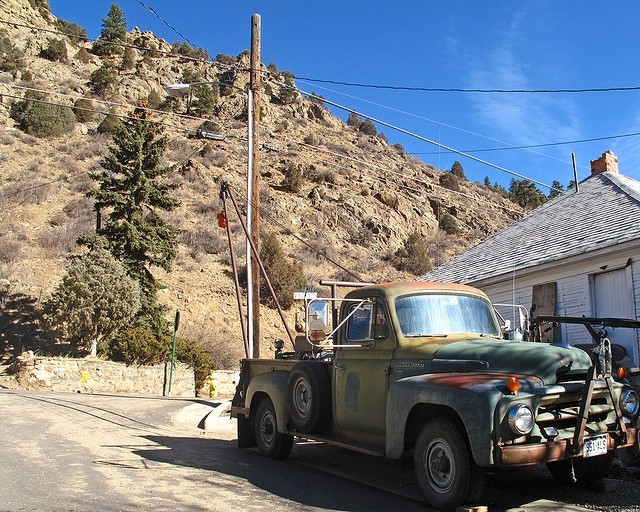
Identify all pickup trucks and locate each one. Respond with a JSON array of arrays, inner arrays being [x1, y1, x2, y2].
[[230, 283, 640, 508]]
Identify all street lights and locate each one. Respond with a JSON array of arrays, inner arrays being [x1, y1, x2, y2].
[[164, 81, 254, 360]]
